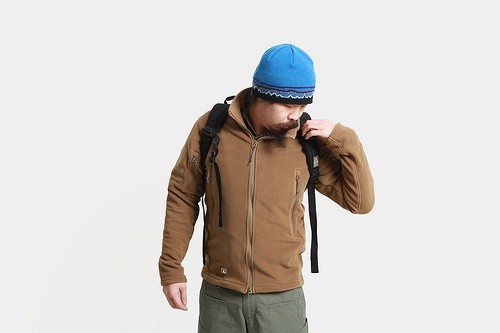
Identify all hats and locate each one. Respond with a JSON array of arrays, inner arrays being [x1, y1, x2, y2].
[[252, 43, 316, 105]]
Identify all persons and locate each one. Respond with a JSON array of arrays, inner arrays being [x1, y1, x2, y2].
[[159, 46, 374, 333]]
